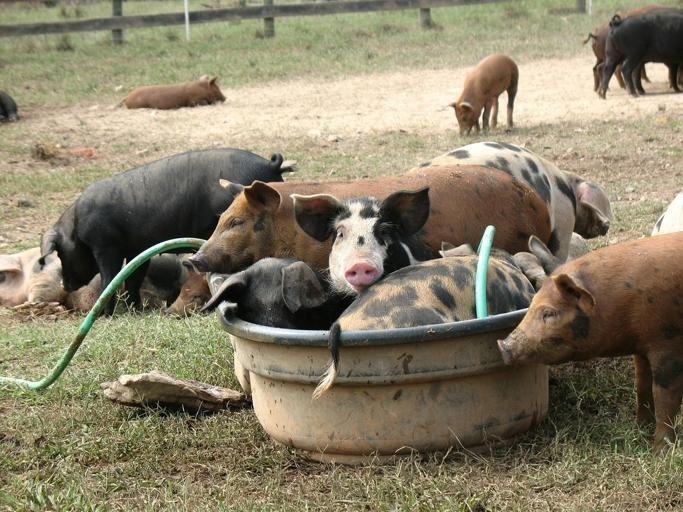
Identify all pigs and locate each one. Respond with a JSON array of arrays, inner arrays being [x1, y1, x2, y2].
[[448, 53, 519, 138], [119, 74, 226, 110], [183, 140, 615, 402], [498, 230, 683, 457], [0, 91, 20, 123], [0, 244, 214, 321], [582, 3, 683, 99], [34, 145, 288, 321]]
